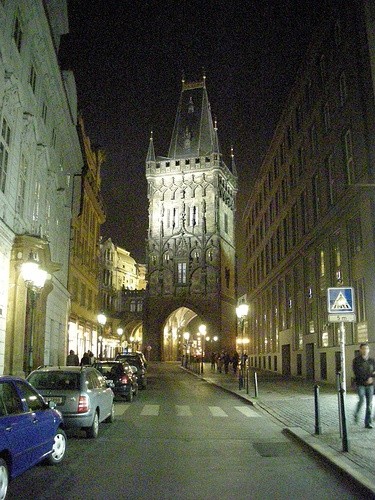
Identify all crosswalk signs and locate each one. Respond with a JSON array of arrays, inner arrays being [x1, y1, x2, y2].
[[327, 286, 355, 314]]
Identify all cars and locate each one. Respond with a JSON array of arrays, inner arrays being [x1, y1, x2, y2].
[[25, 364, 116, 440], [0, 374, 69, 500], [88, 360, 138, 403], [114, 350, 149, 390]]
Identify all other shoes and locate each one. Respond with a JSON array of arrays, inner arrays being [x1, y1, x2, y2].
[[365, 425, 373, 429]]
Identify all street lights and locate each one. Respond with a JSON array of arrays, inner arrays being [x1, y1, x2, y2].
[[234, 303, 249, 389], [97, 312, 107, 361], [117, 327, 123, 354], [199, 324, 207, 374], [135, 337, 141, 351], [130, 336, 135, 353], [183, 332, 190, 368], [19, 249, 49, 379]]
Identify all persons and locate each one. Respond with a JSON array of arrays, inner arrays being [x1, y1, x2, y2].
[[352, 343, 375, 429], [81, 349, 96, 366], [66, 350, 80, 367], [210, 350, 248, 375]]
